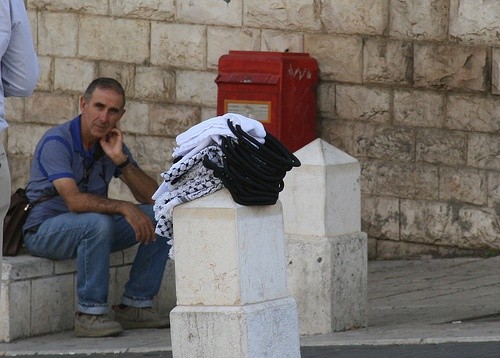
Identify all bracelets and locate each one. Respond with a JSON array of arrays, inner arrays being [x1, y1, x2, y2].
[[116, 155, 130, 170]]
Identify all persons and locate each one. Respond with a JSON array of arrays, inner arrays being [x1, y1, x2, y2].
[[0, 0, 40, 219], [28, 75, 174, 337]]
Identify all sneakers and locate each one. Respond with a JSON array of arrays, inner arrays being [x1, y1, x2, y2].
[[112, 305, 170, 329], [74, 312, 123, 337]]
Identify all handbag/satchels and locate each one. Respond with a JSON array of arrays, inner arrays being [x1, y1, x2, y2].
[[2, 188, 35, 256]]
